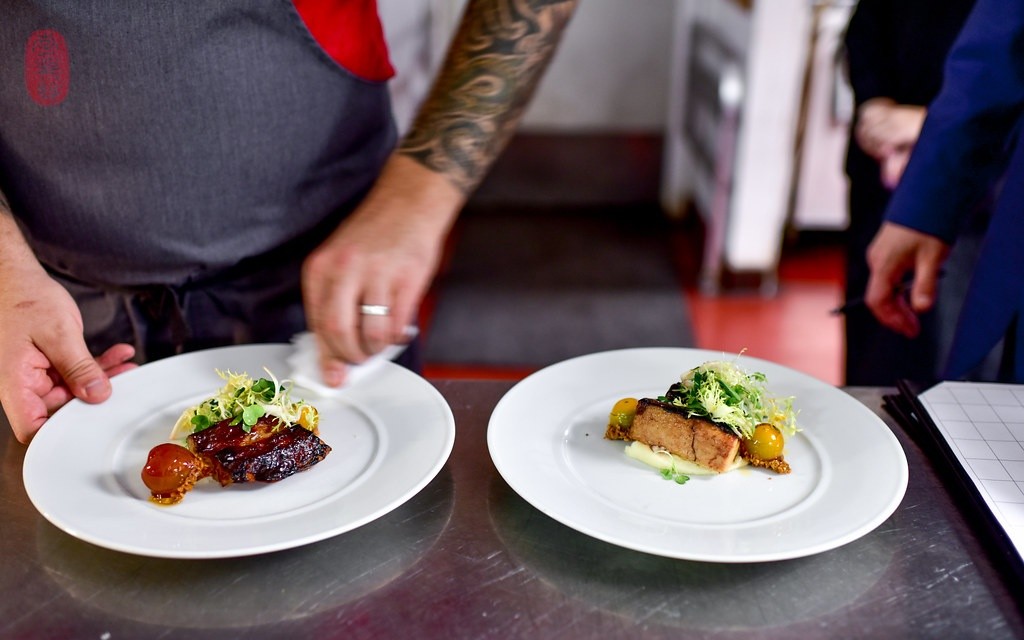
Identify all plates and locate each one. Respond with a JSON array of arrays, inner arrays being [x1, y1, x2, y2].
[[24, 343, 455, 561], [485, 346, 907, 564]]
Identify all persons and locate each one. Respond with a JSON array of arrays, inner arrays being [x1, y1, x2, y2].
[[838, 1, 976, 385], [863, 1, 1022, 386], [0, 0, 583, 447]]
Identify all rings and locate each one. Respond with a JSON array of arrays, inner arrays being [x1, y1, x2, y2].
[[358, 304, 390, 316]]
